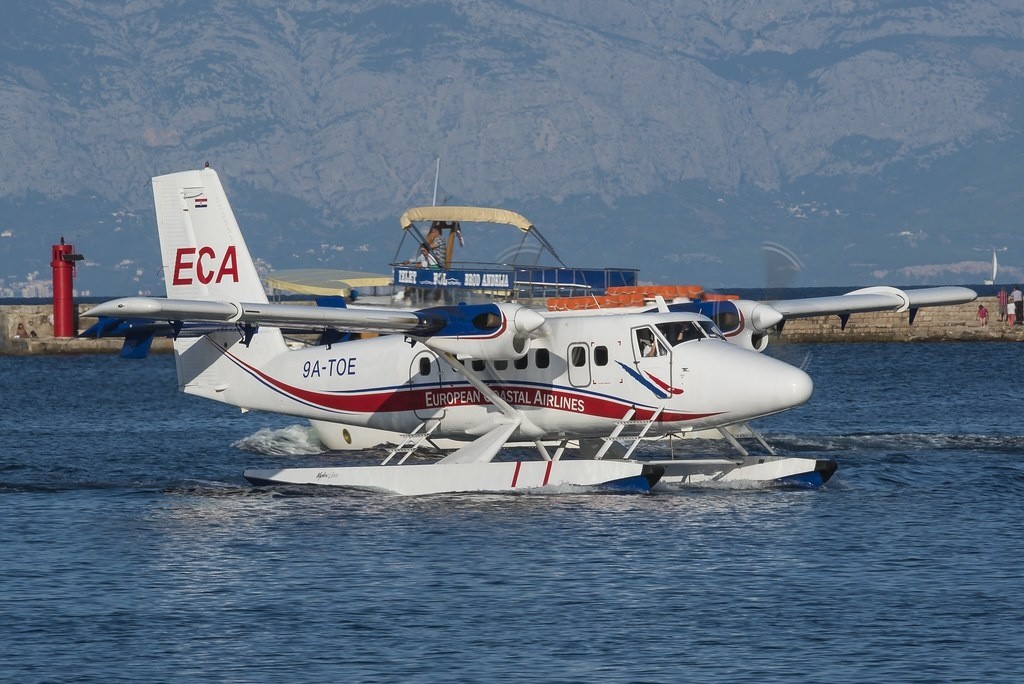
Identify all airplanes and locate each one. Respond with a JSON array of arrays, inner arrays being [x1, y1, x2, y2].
[[76, 152, 979, 496]]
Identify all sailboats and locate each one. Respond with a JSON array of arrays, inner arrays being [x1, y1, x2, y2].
[[984, 251, 998, 285]]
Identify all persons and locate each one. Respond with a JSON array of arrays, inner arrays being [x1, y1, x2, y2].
[[401, 226, 447, 270], [643, 323, 690, 357], [976, 305, 988, 328], [996, 286, 1024, 329], [16, 323, 31, 338]]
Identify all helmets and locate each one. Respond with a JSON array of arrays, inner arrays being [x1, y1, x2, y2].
[[650, 329, 667, 341]]
